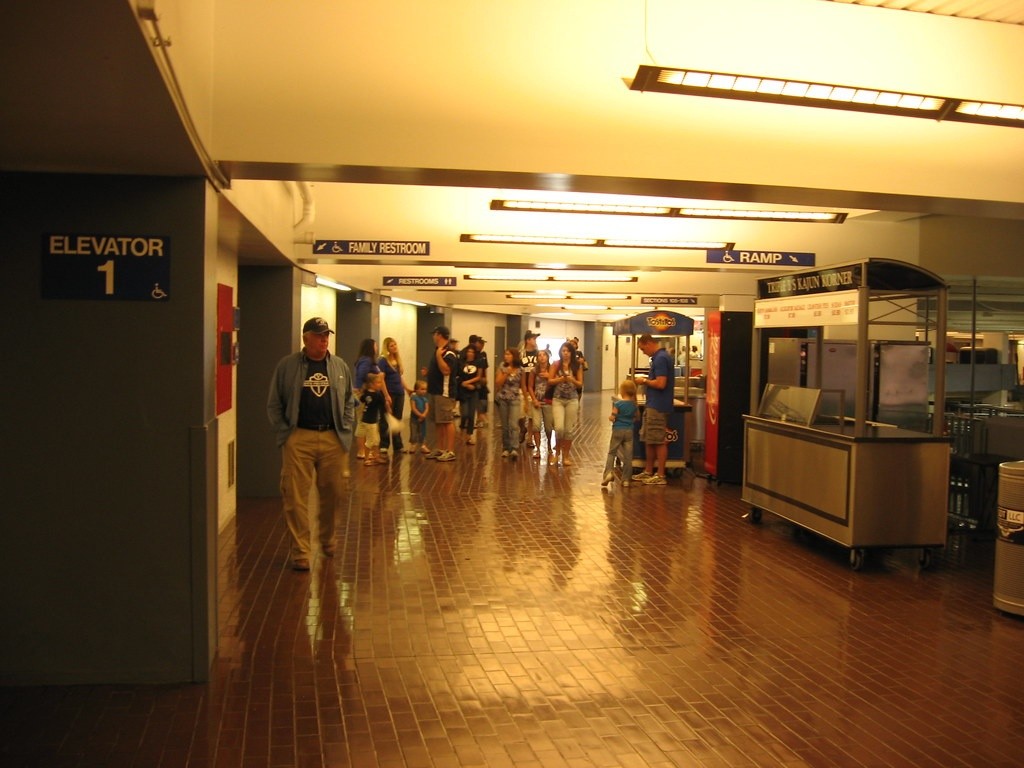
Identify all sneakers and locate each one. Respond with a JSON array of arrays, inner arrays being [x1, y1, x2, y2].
[[631, 472, 653, 480], [293, 558, 310, 571], [323, 535, 337, 557], [641, 472, 667, 485]]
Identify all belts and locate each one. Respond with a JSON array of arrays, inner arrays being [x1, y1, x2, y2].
[[296, 422, 336, 431]]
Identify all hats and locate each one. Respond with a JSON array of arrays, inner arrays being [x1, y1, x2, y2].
[[303, 317, 335, 335], [469, 334, 482, 344], [430, 325, 450, 335], [524, 333, 540, 340], [570, 336, 579, 349], [482, 340, 487, 342], [450, 339, 458, 343]]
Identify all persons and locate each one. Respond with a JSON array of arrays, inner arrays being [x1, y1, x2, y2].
[[667, 345, 701, 377], [354, 338, 393, 458], [408, 378, 431, 455], [447, 330, 589, 466], [601, 380, 642, 488], [353, 370, 393, 466], [264, 314, 356, 574], [631, 333, 675, 485], [376, 337, 414, 455], [421, 326, 460, 462]]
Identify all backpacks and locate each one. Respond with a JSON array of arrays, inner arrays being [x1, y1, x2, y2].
[[436, 344, 469, 385]]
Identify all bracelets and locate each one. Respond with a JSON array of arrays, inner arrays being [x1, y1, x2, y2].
[[643, 379, 647, 384]]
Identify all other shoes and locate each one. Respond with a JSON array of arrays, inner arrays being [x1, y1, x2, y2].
[[601, 471, 614, 486], [358, 420, 573, 466], [623, 481, 630, 487]]
